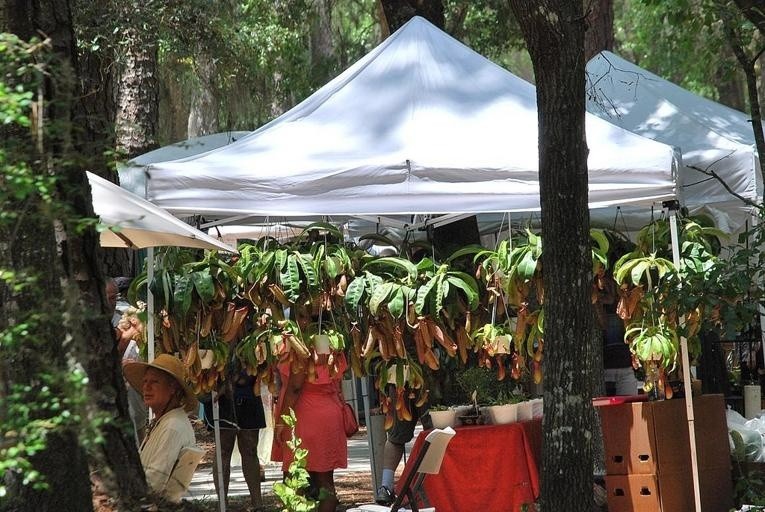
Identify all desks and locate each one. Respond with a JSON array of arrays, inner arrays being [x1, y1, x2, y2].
[[418, 420, 544, 511]]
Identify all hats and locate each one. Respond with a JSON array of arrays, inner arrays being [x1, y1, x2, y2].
[[123, 354, 197, 412]]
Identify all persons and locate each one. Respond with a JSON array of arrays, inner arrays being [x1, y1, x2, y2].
[[603, 302, 638, 396]]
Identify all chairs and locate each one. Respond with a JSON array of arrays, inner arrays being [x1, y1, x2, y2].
[[345, 425, 458, 510], [162, 445, 210, 500]]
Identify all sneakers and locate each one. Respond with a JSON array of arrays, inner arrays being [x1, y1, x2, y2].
[[377, 487, 396, 504]]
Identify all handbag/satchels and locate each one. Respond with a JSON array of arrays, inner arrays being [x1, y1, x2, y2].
[[203, 392, 258, 433], [341, 404, 359, 438]]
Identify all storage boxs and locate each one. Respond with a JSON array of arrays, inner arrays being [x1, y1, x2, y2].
[[601, 390, 735, 512]]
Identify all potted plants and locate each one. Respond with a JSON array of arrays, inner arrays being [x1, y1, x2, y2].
[[428, 364, 544, 427]]
